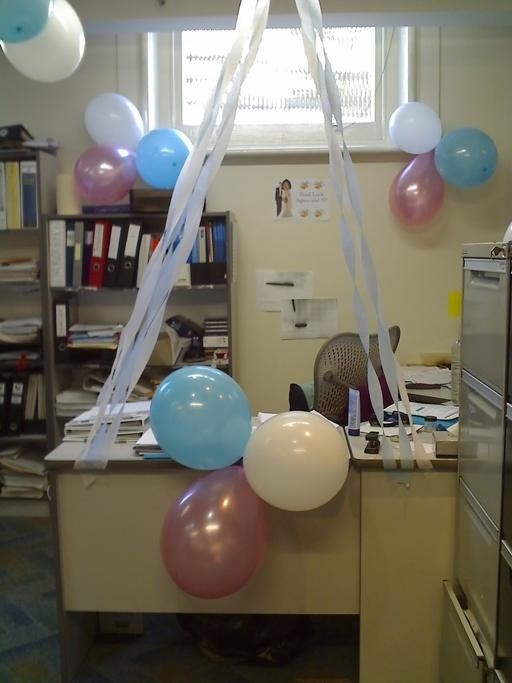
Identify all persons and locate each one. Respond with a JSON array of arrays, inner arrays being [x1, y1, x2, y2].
[[275, 182, 282, 216], [279, 179, 292, 217]]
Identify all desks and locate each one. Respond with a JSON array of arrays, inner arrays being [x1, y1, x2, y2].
[[44, 423, 360, 683], [345, 366, 461, 682]]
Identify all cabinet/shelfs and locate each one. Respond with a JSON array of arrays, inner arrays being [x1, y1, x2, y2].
[[440, 240, 511, 682], [45, 210, 236, 442], [1, 141, 58, 520]]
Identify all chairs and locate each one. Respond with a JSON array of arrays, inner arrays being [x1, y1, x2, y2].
[[290, 382, 317, 412]]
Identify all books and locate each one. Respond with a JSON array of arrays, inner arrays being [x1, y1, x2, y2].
[[0, 318, 42, 345], [0, 259, 41, 283], [133, 428, 169, 459], [0, 445, 49, 499], [66, 323, 118, 350]]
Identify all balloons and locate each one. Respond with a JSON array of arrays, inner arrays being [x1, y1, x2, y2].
[[0, 0, 86, 83], [74, 143, 136, 206], [160, 465, 268, 600], [242, 411, 350, 512], [434, 127, 498, 189], [83, 92, 145, 149], [135, 127, 194, 189], [149, 365, 252, 472], [389, 153, 444, 228], [388, 101, 442, 155], [0, 0, 51, 43]]
